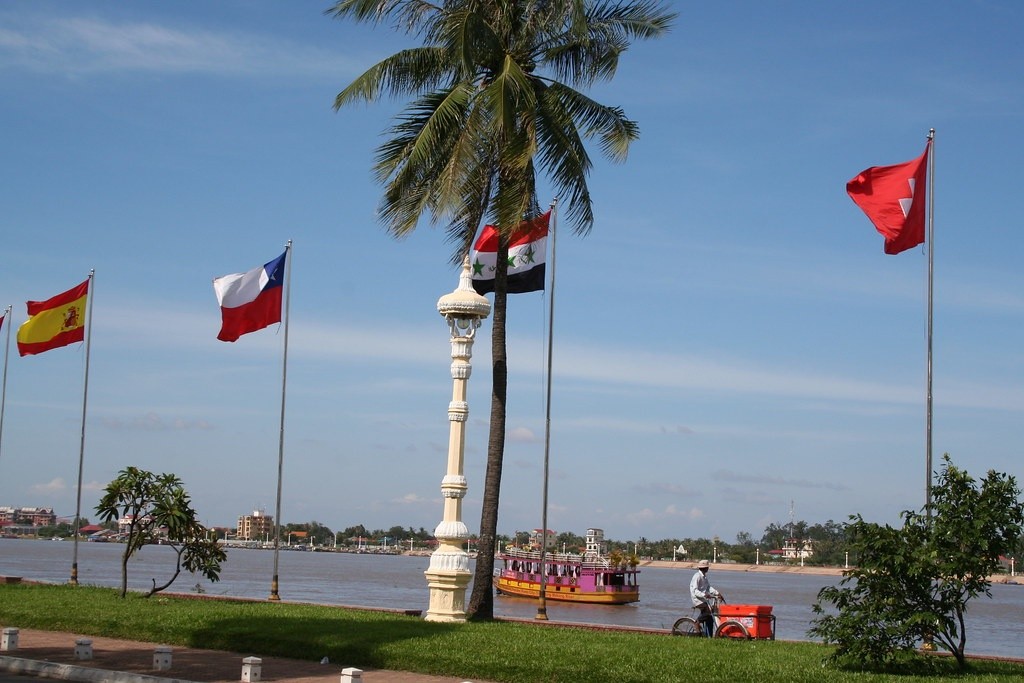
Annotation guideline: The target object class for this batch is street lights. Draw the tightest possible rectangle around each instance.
[497,540,502,553]
[385,537,387,550]
[266,533,269,543]
[563,542,566,555]
[359,536,361,548]
[334,535,337,547]
[419,256,492,635]
[310,536,313,546]
[288,533,291,547]
[713,547,717,564]
[801,549,804,567]
[673,545,676,562]
[411,537,414,552]
[225,532,227,541]
[467,539,470,553]
[756,548,759,566]
[634,544,638,556]
[845,551,849,569]
[1011,557,1015,577]
[206,530,208,540]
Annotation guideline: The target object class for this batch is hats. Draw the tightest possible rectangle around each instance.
[697,560,711,569]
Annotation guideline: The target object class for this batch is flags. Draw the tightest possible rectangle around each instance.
[213,247,288,342]
[471,206,552,297]
[846,139,930,256]
[17,278,90,357]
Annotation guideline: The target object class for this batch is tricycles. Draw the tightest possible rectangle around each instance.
[670,594,776,641]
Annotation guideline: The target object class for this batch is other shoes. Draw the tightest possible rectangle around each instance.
[694,621,701,634]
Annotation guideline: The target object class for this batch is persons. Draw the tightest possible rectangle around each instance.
[690,559,722,638]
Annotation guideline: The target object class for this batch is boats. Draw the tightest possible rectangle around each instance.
[491,542,643,605]
